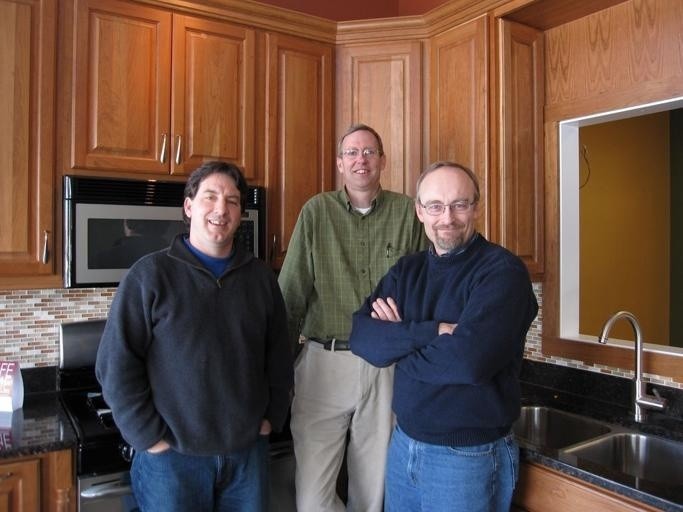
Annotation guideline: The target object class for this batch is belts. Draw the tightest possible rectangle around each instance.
[319,339,353,351]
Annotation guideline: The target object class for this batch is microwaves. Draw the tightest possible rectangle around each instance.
[61,175,267,290]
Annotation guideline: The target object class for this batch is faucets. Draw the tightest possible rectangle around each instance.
[598,309,667,422]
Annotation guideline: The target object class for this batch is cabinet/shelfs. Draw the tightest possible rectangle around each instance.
[3,0,61,290]
[264,5,541,280]
[0,391,80,512]
[57,0,264,179]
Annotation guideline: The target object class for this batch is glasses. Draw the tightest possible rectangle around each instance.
[419,201,474,216]
[342,149,382,158]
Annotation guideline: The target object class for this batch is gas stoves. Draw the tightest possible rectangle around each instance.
[59,386,136,479]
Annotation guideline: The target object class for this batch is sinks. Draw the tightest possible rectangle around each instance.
[512,405,621,452]
[563,432,683,505]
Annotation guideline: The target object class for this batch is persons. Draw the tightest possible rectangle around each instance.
[276,124,433,511]
[347,160,537,512]
[95,162,294,512]
